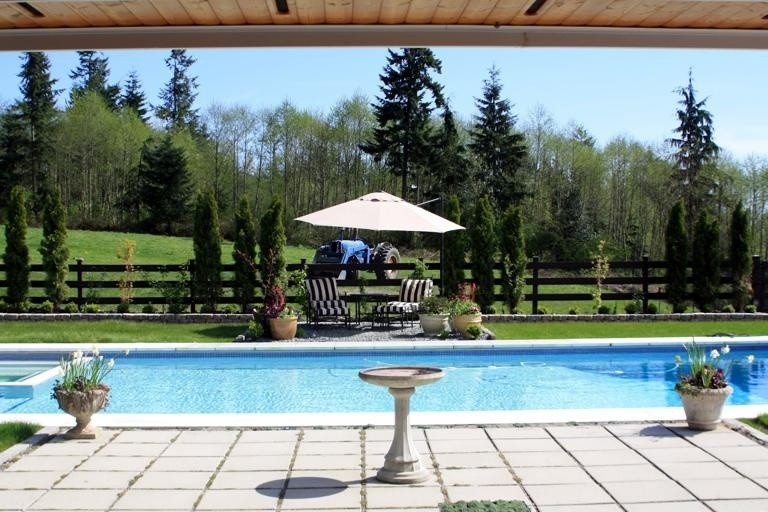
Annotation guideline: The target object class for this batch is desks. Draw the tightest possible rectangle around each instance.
[357,366,448,485]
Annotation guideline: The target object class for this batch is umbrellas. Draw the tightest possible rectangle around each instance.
[293,190,466,235]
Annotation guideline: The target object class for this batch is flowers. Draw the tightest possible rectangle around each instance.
[259,283,299,319]
[58,347,117,390]
[419,281,483,316]
[673,344,754,389]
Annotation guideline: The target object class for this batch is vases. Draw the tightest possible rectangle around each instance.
[54,385,110,440]
[417,313,482,339]
[675,382,732,431]
[253,307,300,343]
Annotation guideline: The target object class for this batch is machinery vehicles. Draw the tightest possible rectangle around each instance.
[304,228,400,280]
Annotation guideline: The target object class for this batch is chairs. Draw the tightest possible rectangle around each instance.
[304,277,435,332]
